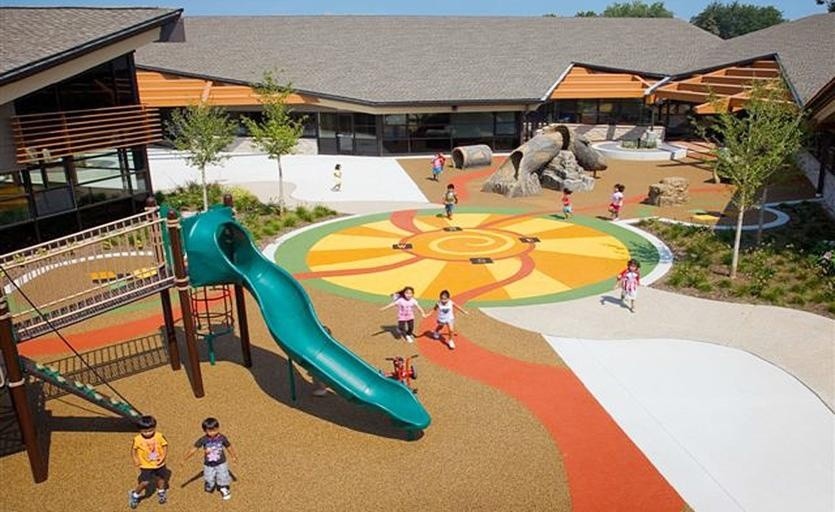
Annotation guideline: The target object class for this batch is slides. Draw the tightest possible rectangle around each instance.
[159,203,430,439]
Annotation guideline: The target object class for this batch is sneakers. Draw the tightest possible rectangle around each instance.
[609,210,620,222]
[398,334,415,343]
[447,340,458,350]
[445,210,454,220]
[432,330,441,340]
[155,489,169,507]
[207,485,232,500]
[126,489,141,511]
[564,213,575,221]
[622,298,638,313]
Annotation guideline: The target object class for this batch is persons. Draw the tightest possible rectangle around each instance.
[426,290,468,350]
[442,184,458,219]
[131,416,169,509]
[331,164,341,191]
[431,152,446,182]
[616,259,641,312]
[312,326,331,396]
[561,188,574,221]
[608,184,624,221]
[180,417,237,500]
[379,285,426,344]
